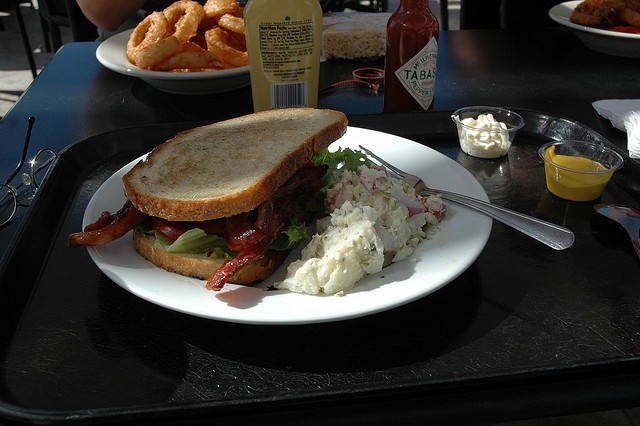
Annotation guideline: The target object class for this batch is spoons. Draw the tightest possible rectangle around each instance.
[594,202,639,260]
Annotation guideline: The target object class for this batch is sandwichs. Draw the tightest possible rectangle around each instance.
[66,105,349,292]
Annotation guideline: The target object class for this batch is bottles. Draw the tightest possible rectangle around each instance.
[384,0,438,115]
[244,0,323,113]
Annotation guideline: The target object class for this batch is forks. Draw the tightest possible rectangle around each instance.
[354,144,575,251]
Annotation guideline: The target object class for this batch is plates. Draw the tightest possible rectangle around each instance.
[83,126,494,327]
[548,0,640,59]
[96,26,250,95]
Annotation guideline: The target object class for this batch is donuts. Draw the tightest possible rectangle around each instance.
[126,0,249,73]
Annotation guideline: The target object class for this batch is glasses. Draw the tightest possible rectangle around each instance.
[0,116,57,226]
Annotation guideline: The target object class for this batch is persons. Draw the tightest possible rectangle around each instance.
[75,0,184,41]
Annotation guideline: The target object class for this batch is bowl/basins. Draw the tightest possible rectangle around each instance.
[451,106,524,159]
[538,140,625,201]
